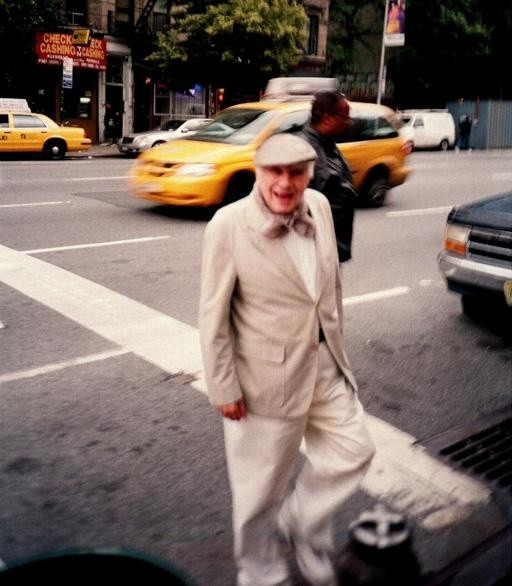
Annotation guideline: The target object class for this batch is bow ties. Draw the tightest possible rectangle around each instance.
[261,213,318,241]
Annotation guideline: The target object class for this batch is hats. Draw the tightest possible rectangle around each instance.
[254,134,318,170]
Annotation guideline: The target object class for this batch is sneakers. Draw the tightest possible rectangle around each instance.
[278,515,335,584]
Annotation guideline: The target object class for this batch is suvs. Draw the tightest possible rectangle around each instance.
[438,191,512,323]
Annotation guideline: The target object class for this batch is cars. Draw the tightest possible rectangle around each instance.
[1,95,92,161]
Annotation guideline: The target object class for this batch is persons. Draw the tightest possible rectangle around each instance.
[191,128,379,586]
[101,101,119,148]
[289,89,362,353]
